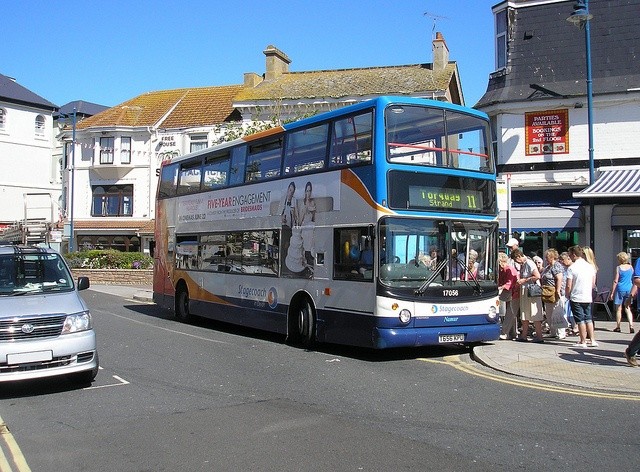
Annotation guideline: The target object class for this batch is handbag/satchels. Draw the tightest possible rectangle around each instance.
[528,277,542,297]
[499,289,513,302]
[541,284,556,303]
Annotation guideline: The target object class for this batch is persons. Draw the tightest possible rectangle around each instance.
[505,237,520,252]
[532,255,545,274]
[565,245,599,347]
[480,251,487,270]
[559,252,581,337]
[359,231,388,265]
[540,248,566,341]
[276,182,298,255]
[624,256,640,367]
[460,254,479,280]
[497,252,521,339]
[409,245,460,279]
[510,248,544,343]
[457,245,467,264]
[575,246,598,344]
[608,252,638,333]
[468,249,480,270]
[298,181,316,251]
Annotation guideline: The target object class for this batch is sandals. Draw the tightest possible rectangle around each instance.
[614,328,620,332]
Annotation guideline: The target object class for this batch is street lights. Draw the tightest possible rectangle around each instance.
[566,0,595,252]
[57,108,77,254]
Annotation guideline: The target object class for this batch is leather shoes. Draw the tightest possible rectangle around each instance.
[624,349,638,367]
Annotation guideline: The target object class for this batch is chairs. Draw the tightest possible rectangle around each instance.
[592,288,614,320]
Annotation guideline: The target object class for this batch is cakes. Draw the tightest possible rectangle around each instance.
[285,227,307,272]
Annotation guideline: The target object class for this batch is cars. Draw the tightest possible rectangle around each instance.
[0,246,98,383]
[175,241,198,256]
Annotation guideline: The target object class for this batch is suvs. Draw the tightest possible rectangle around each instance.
[200,262,276,276]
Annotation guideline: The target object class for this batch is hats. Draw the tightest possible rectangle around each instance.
[506,238,519,247]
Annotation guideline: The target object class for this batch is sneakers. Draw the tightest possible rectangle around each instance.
[499,334,512,339]
[587,342,599,347]
[572,343,587,348]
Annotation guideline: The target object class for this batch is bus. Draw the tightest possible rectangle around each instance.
[153,96,524,350]
